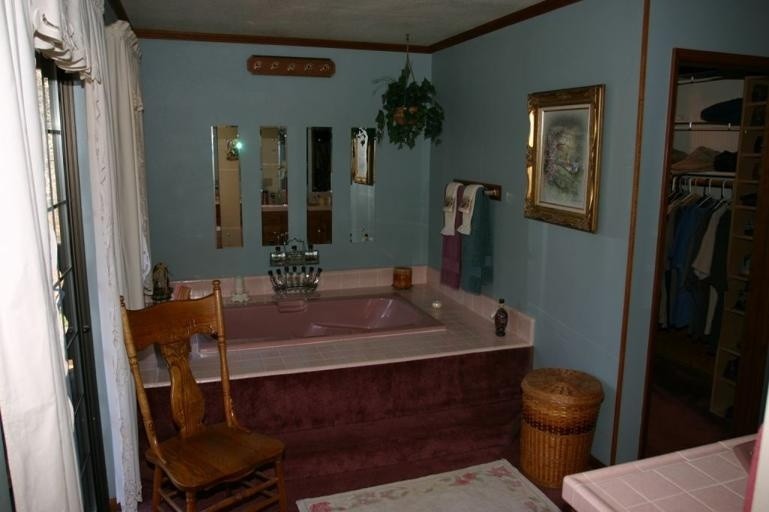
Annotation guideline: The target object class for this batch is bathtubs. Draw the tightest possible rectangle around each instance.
[196,292,448,355]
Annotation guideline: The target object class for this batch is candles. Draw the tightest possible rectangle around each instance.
[119,279,285,512]
[233,275,248,292]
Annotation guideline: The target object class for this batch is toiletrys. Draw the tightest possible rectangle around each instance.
[268,245,323,294]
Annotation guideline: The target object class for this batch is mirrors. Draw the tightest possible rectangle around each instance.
[209,123,333,250]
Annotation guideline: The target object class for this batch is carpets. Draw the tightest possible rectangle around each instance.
[293,458,562,512]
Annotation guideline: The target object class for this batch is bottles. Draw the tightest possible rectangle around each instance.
[269,244,319,266]
[268,266,323,294]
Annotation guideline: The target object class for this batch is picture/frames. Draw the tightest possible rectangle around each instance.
[523,84,606,235]
[352,130,373,187]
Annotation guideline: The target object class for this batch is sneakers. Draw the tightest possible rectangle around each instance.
[671,146,721,174]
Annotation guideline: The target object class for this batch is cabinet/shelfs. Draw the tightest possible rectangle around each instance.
[707,75,767,425]
[668,121,739,189]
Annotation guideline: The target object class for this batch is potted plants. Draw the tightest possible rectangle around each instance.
[368,29,445,148]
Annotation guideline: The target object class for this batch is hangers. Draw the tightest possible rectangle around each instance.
[660,172,734,221]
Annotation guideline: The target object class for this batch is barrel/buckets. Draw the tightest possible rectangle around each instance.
[393,267,411,289]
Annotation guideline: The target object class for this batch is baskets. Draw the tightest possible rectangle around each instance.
[520,368,604,488]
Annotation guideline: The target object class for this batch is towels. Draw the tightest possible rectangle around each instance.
[436,182,487,290]
